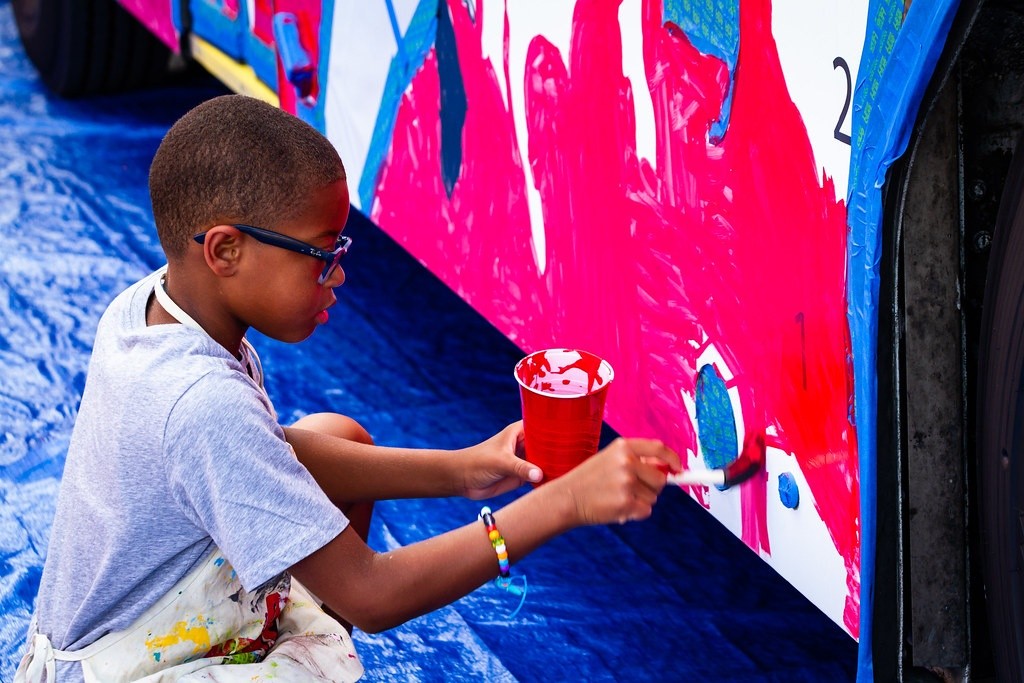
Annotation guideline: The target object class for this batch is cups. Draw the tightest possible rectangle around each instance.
[514,347,615,490]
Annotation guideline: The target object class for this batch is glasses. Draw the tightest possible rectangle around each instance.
[194,225,352,285]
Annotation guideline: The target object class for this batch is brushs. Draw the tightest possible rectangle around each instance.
[665,446,760,488]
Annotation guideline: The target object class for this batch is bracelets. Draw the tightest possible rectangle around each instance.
[482,505,511,576]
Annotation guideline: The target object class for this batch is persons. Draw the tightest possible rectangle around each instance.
[14,93,684,683]
